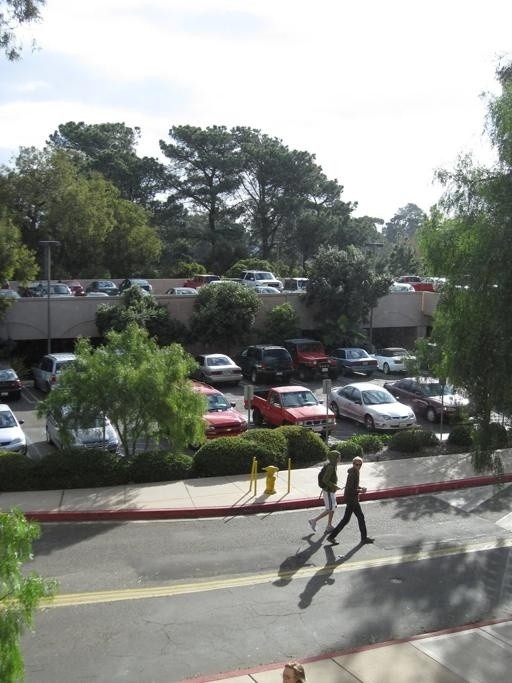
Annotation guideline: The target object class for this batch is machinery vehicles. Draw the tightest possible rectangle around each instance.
[369,346,417,373]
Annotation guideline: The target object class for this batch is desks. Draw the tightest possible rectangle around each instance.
[363,242,384,344]
[38,239,62,352]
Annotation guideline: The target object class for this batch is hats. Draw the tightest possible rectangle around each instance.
[324,381,416,432]
[382,374,475,422]
[44,400,120,454]
[0,360,22,400]
[0,402,27,455]
[193,352,244,386]
[328,347,379,376]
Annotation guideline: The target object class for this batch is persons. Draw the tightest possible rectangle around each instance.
[307,450,341,532]
[326,455,376,543]
[282,662,307,683]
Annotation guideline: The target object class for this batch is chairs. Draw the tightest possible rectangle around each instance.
[326,525,335,532]
[326,535,339,544]
[362,537,375,543]
[308,518,316,531]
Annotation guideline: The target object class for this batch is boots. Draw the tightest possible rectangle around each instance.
[234,344,295,383]
[184,377,248,449]
[32,352,88,392]
[283,338,338,381]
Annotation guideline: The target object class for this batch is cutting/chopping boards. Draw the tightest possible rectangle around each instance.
[262,466,280,494]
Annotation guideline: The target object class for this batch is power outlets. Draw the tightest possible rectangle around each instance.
[244,385,337,436]
